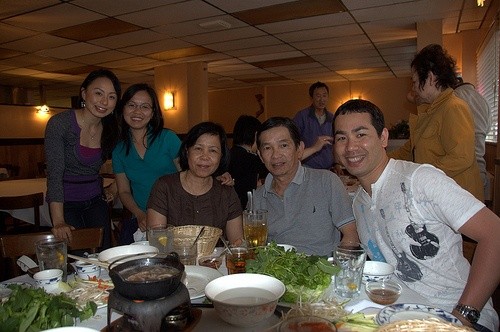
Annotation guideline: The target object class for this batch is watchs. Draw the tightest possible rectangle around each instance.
[456,305,480,323]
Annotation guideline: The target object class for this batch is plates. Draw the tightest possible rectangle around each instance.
[280,314,338,332]
[267,243,298,253]
[376,302,464,328]
[97,243,159,263]
[180,264,223,300]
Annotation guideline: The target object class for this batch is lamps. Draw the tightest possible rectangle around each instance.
[37,103,53,115]
[163,89,175,111]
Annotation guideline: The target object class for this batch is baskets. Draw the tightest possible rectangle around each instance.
[166,225,223,256]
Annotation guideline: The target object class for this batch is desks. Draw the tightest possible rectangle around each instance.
[0,178,54,227]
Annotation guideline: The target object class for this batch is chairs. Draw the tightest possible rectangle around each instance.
[0,191,44,235]
[0,226,105,257]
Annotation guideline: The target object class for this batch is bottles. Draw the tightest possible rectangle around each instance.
[148,224,173,254]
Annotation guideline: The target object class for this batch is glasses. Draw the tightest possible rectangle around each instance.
[125,100,154,114]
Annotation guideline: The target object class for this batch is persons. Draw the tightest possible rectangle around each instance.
[245,117,359,257]
[292,82,335,169]
[407,44,491,206]
[332,99,500,332]
[44,69,122,257]
[227,115,269,212]
[111,83,182,246]
[146,121,245,248]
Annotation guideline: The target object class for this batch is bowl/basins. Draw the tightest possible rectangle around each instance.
[108,251,185,301]
[363,260,395,281]
[33,268,63,288]
[204,274,286,326]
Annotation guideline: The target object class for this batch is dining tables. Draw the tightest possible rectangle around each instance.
[0,247,493,332]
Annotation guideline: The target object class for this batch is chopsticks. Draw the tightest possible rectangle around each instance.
[66,252,116,270]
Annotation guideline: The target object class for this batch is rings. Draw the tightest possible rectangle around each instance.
[324,139,326,141]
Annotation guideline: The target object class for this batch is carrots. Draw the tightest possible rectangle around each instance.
[90,276,112,302]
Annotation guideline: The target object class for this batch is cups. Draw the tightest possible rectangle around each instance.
[175,244,198,265]
[333,241,367,298]
[37,238,68,274]
[242,208,269,247]
[199,256,222,270]
[224,248,249,273]
[366,280,403,304]
[69,256,101,279]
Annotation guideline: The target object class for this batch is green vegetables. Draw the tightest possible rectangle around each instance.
[245,239,339,306]
[232,257,244,263]
[0,283,98,332]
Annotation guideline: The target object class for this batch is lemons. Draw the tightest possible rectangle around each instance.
[158,236,167,248]
[56,251,63,265]
